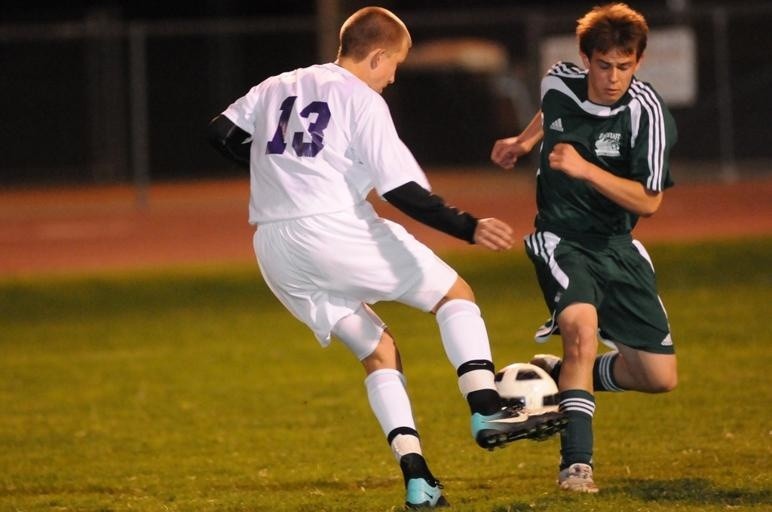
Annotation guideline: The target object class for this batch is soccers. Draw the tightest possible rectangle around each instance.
[495,363,559,411]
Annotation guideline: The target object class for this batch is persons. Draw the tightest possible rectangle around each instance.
[490,2,679,492]
[205,6,569,511]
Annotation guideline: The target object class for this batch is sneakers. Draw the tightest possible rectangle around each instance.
[529,353,561,376]
[470,401,561,451]
[405,478,449,510]
[557,456,599,494]
